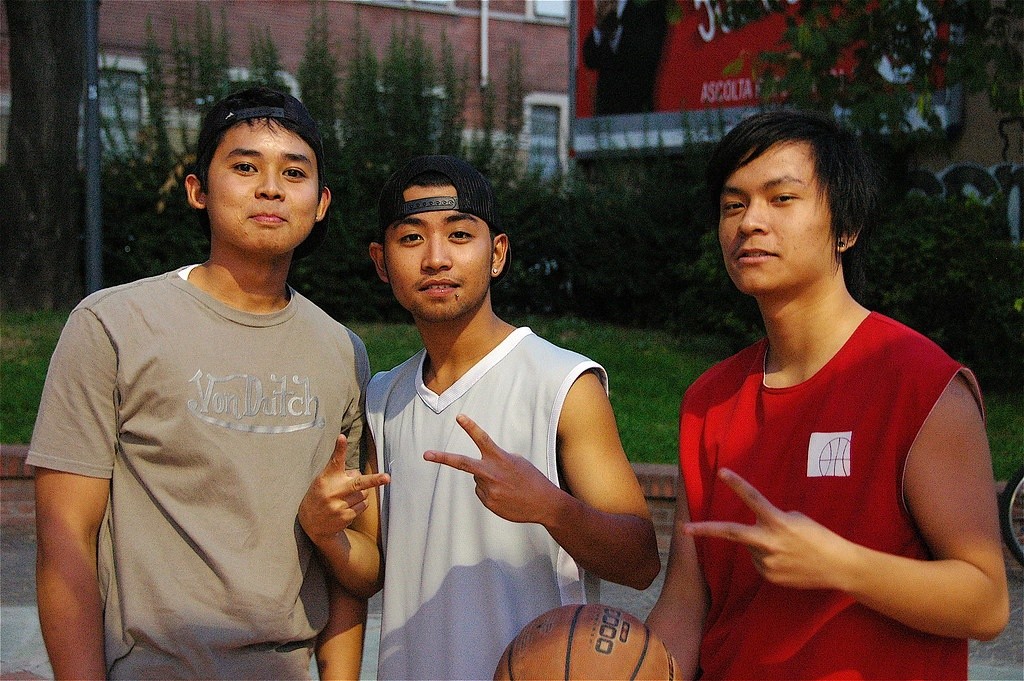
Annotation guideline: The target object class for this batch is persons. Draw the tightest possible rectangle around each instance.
[23,86,373,681]
[642,112,1013,681]
[580,1,669,117]
[296,153,662,681]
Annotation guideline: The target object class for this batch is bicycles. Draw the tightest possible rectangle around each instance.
[999,463,1024,566]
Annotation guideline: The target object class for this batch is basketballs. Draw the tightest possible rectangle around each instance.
[492,603,681,681]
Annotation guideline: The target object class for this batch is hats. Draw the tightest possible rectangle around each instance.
[198,88,329,260]
[379,155,511,287]
